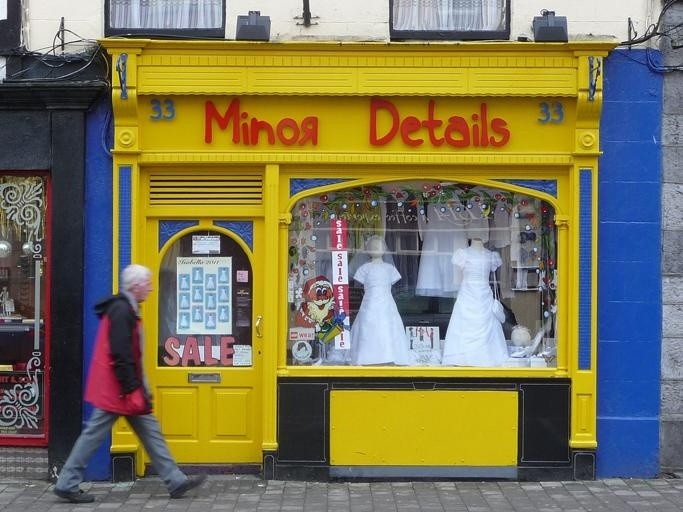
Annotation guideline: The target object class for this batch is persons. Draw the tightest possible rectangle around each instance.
[54,264,207,503]
[452,239,502,364]
[0,286,14,316]
[352,235,401,363]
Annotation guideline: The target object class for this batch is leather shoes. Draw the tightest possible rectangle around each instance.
[170,473,206,500]
[52,489,99,504]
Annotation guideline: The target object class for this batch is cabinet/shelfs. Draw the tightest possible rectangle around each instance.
[508,193,541,345]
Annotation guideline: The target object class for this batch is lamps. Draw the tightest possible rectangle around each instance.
[533,11,568,42]
[236,11,271,41]
